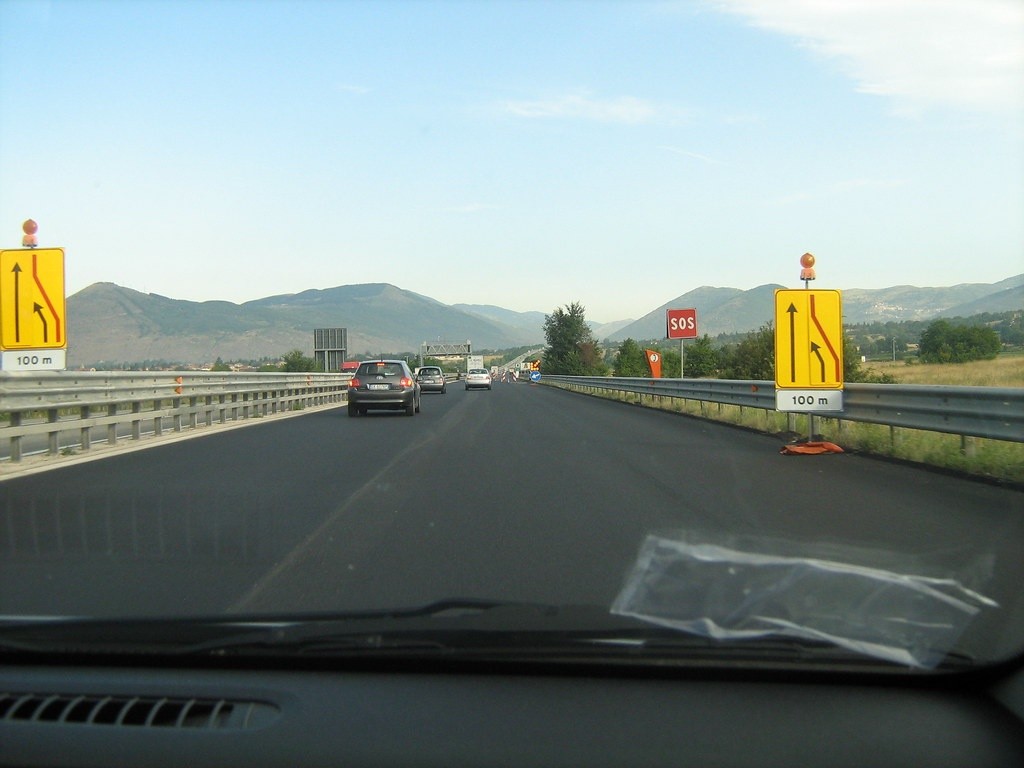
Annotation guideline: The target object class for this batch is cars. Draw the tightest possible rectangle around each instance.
[463,368,492,391]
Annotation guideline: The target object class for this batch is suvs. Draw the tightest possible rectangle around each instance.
[347,360,422,416]
[416,365,447,394]
